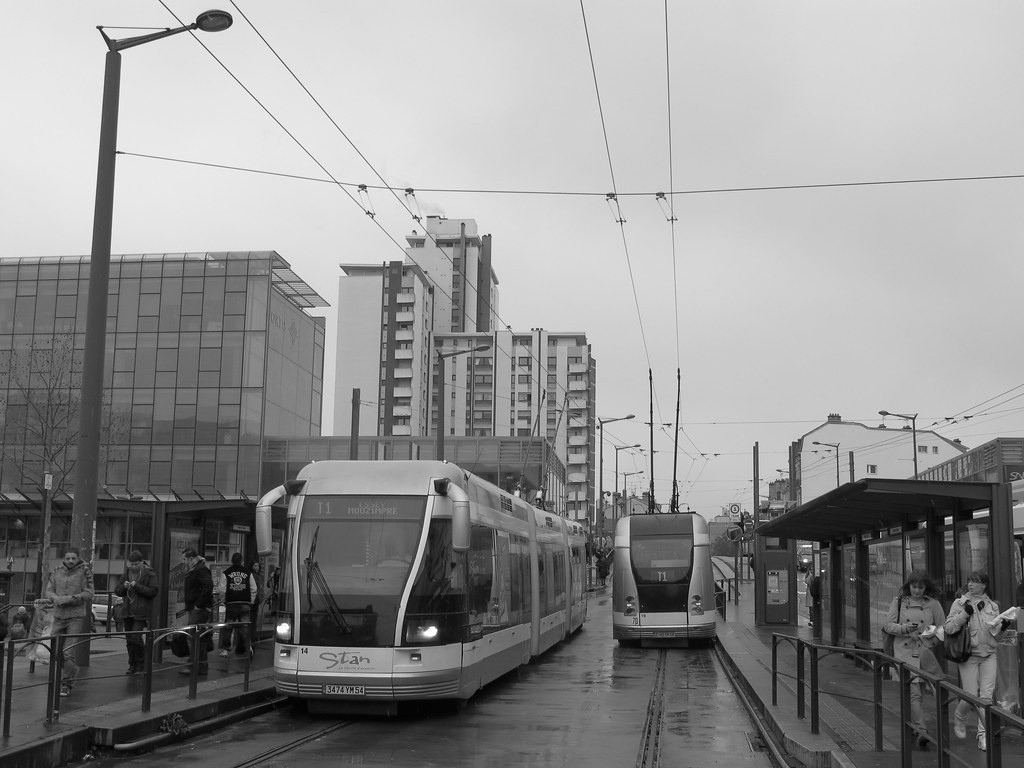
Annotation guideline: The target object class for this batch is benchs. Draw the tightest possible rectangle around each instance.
[839,638,889,679]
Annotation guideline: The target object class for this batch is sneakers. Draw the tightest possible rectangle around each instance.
[953,714,966,740]
[976,732,986,751]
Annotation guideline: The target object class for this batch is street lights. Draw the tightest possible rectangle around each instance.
[614,444,641,519]
[812,441,841,488]
[879,410,918,478]
[72,6,235,671]
[597,414,635,556]
[622,471,645,515]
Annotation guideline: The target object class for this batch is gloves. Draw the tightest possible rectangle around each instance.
[1001,619,1010,632]
[964,603,974,615]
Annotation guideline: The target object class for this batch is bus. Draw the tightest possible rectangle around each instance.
[610,512,719,647]
[254,459,589,711]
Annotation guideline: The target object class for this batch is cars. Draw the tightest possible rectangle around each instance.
[797,544,813,570]
[86,595,120,624]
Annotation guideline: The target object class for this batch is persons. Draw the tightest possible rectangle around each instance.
[114,550,159,675]
[804,564,814,627]
[90,612,97,634]
[376,532,414,567]
[945,571,1011,751]
[883,568,946,747]
[12,606,31,644]
[178,548,214,681]
[47,548,94,696]
[218,553,261,657]
[585,535,614,585]
[113,597,125,632]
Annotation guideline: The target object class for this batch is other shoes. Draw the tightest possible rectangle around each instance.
[178,667,209,676]
[220,649,228,658]
[912,729,930,748]
[808,621,814,627]
[60,686,69,696]
[126,664,145,674]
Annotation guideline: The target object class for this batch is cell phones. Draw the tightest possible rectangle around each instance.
[912,623,918,628]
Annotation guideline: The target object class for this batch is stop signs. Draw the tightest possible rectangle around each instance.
[745,520,754,528]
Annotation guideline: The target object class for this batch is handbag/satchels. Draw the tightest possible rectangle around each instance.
[882,627,896,658]
[942,602,973,663]
[170,626,214,658]
